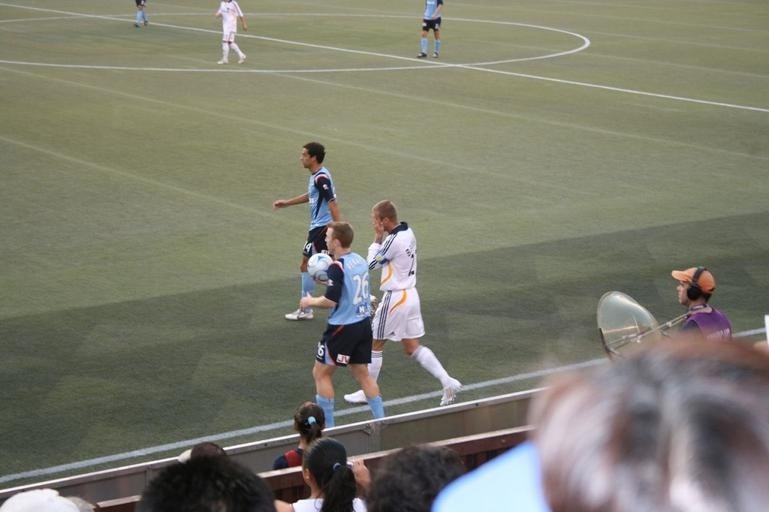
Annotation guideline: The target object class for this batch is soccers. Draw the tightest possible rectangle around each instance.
[307,253,333,281]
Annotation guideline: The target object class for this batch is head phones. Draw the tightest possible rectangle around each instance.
[688,266,712,300]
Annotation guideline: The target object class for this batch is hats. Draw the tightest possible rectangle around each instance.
[671,265,716,297]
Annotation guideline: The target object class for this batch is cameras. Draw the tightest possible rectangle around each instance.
[346,462,356,474]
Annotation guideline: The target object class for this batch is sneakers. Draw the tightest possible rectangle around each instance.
[217,60,229,64]
[415,52,427,57]
[283,308,314,321]
[237,55,247,64]
[432,50,439,58]
[342,389,368,406]
[439,379,464,408]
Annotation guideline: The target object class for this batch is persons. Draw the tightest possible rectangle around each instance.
[215,1,248,65]
[297,223,388,425]
[671,266,733,343]
[274,144,377,324]
[134,1,148,28]
[341,197,463,408]
[417,0,444,60]
